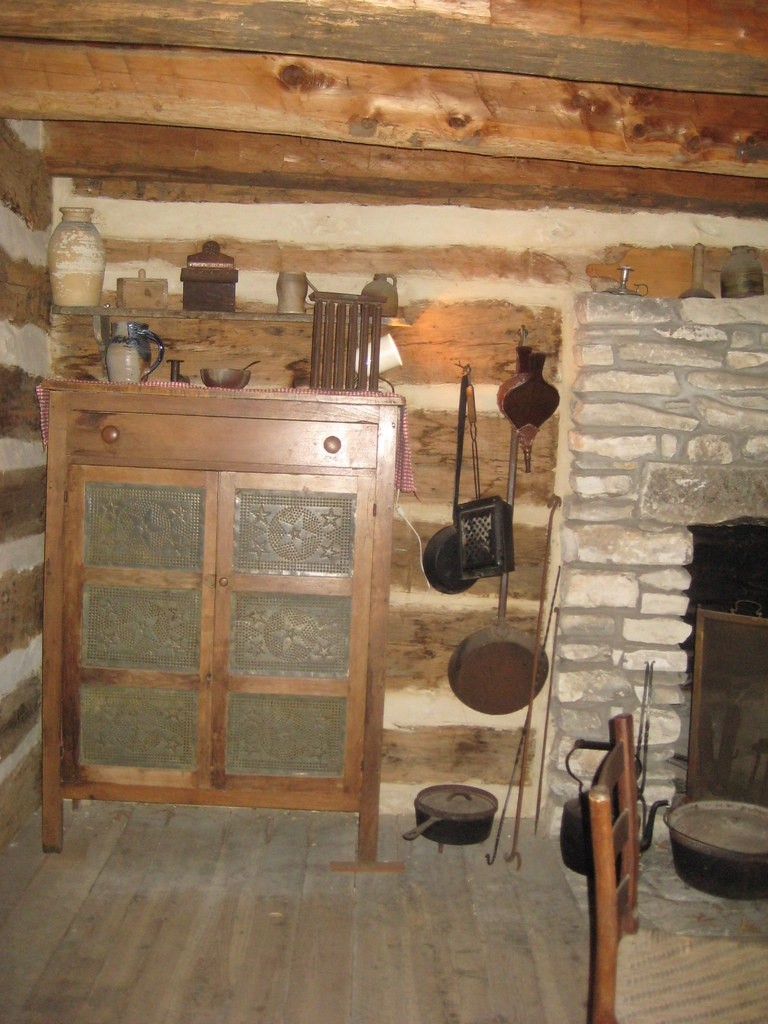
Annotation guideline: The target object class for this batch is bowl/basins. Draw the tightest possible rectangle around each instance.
[200,368,251,389]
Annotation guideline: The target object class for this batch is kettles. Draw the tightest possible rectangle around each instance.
[559,739,669,876]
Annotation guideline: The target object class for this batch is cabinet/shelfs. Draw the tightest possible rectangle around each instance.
[39,381,404,866]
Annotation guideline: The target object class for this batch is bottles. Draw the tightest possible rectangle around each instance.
[47,207,106,307]
[361,273,398,317]
[275,271,308,314]
[720,245,764,299]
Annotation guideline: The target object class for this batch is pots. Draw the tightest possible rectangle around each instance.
[402,783,498,846]
[663,799,768,902]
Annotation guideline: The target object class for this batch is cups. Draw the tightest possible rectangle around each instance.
[355,333,402,378]
[104,321,165,383]
